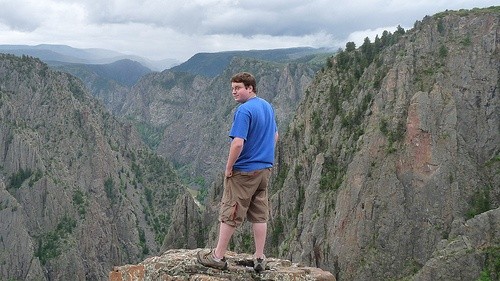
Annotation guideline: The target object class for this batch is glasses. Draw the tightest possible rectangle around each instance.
[231,85,246,90]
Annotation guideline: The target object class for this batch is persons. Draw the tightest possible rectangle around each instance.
[196,73,279,272]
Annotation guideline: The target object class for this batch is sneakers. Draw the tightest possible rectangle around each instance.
[197,248,227,270]
[253,253,266,271]
